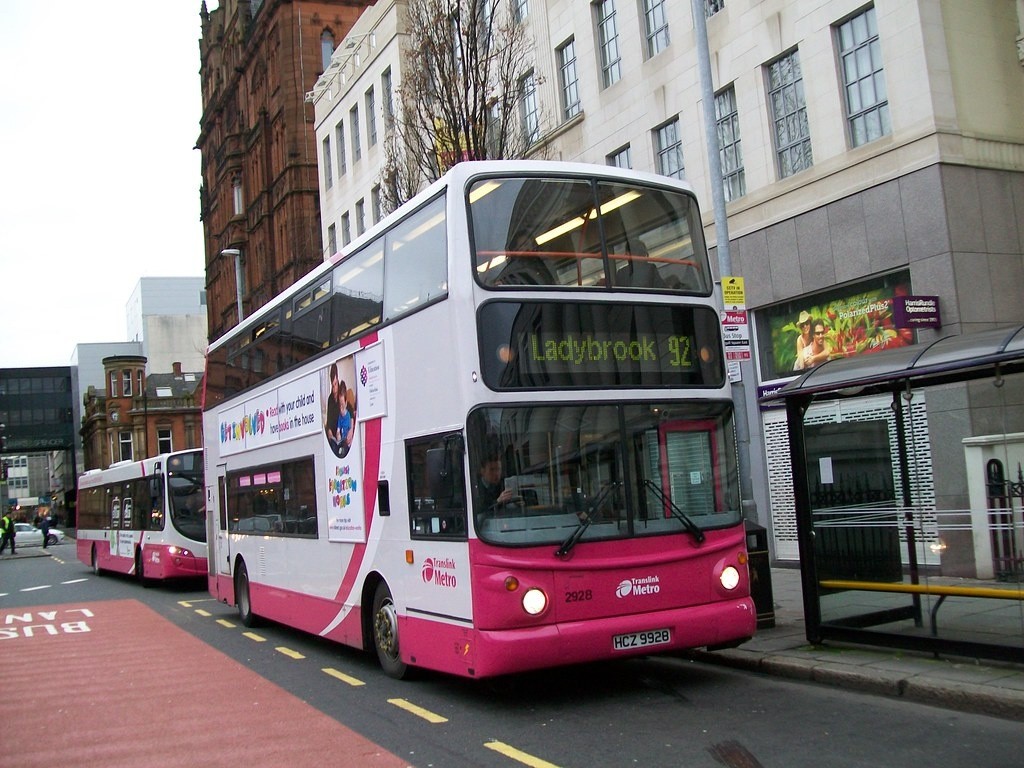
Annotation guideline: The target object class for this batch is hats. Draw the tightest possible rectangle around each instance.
[796,311,813,327]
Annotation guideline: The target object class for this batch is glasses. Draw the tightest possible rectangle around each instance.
[814,332,824,335]
[800,320,809,328]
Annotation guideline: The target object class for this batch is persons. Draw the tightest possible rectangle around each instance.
[326,364,354,457]
[616,239,667,287]
[793,311,831,372]
[0,512,18,554]
[492,230,561,285]
[473,455,525,530]
[14,512,50,550]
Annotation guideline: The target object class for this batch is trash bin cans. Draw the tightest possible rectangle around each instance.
[744,520,776,629]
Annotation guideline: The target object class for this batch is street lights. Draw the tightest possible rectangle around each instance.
[219,247,245,330]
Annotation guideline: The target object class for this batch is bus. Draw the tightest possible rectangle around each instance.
[76,448,207,584]
[200,160,760,682]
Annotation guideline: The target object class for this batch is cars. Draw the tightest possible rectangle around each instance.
[44,514,59,527]
[4,523,66,547]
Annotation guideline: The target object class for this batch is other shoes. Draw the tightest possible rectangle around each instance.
[11,552,17,555]
